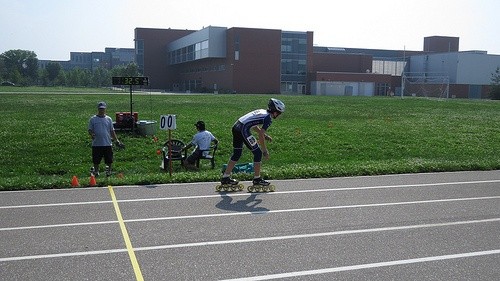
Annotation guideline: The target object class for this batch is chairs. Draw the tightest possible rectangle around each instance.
[163,139,187,169]
[197,140,219,169]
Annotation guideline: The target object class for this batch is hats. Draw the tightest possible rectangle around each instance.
[98,102,106,109]
[194,121,205,128]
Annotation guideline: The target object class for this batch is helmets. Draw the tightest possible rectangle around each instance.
[269,97,285,113]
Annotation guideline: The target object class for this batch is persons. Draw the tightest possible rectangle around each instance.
[88,102,120,177]
[177,121,218,171]
[216,98,285,192]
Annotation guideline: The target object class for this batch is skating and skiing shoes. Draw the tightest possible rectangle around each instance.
[247,175,275,192]
[215,176,244,192]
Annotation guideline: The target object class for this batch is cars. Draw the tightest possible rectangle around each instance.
[1,82,15,86]
